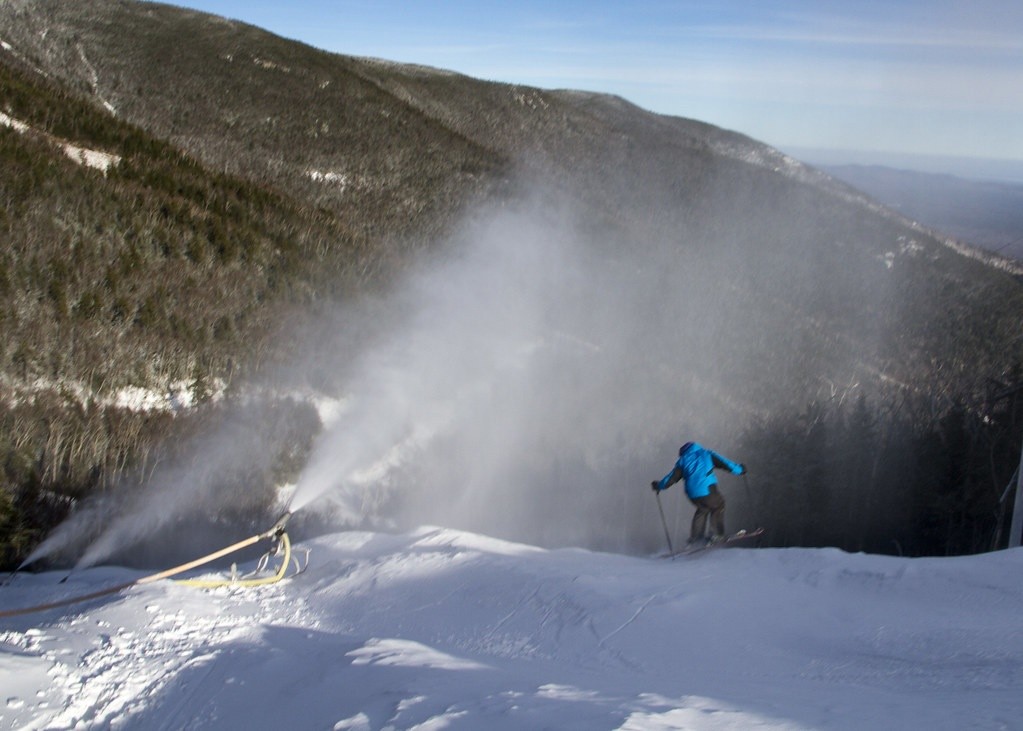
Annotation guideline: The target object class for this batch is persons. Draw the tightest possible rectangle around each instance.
[653,442,748,548]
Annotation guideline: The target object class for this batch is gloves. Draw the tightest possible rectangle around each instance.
[651,480,661,495]
[740,464,747,474]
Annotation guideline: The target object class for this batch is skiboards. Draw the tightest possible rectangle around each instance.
[639,526,766,558]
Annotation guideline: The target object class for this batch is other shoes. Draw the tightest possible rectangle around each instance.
[709,535,729,543]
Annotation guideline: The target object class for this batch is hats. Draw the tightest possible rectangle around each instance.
[679,442,693,456]
